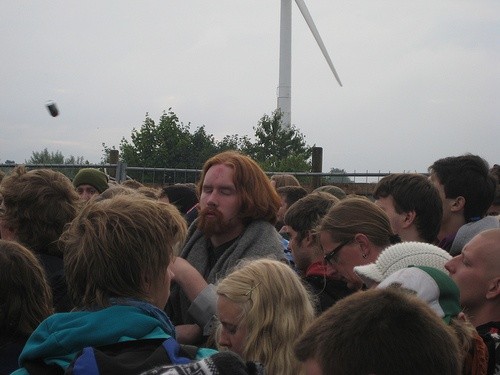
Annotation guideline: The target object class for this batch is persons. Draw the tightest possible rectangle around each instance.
[0,150,500,375]
[292,287,461,375]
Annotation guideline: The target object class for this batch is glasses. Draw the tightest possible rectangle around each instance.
[324,241,346,265]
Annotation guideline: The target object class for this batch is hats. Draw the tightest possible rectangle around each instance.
[71,167,110,193]
[373,264,462,326]
[449,215,499,256]
[164,186,197,214]
[354,242,454,289]
